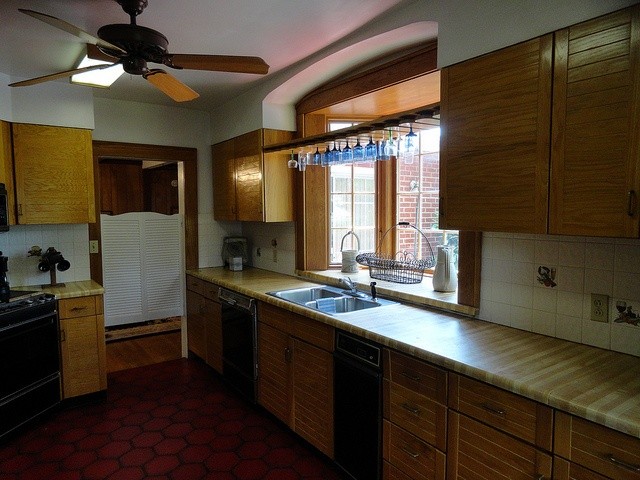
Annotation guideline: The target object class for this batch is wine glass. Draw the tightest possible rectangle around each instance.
[365,136,376,159]
[384,128,397,157]
[314,147,321,164]
[354,139,364,159]
[307,147,314,165]
[324,145,331,161]
[396,124,405,159]
[298,149,306,172]
[288,150,297,169]
[343,141,352,160]
[379,141,389,158]
[406,127,417,148]
[331,143,338,161]
[403,133,413,165]
[338,145,342,160]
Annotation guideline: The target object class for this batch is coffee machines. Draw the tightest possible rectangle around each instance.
[224,236,251,271]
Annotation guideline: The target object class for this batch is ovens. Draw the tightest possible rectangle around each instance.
[217,287,259,402]
[0,294,60,441]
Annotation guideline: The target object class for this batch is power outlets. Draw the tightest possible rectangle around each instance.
[589,294,609,323]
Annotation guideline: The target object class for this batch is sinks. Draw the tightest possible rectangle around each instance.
[306,297,382,314]
[275,288,343,304]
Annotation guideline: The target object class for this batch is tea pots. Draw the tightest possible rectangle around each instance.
[432,245,457,292]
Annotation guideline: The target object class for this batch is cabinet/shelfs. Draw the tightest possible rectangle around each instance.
[552,408,639,479]
[381,349,448,478]
[10,123,96,225]
[447,374,555,480]
[549,2,640,240]
[0,117,16,230]
[439,33,553,235]
[210,129,295,224]
[254,302,334,459]
[58,295,109,400]
[185,273,223,372]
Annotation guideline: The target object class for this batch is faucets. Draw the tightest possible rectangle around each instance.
[338,277,357,294]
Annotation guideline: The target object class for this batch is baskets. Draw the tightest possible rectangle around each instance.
[341,230,360,274]
[355,222,436,285]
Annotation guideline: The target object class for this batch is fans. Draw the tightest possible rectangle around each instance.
[9,1,269,103]
